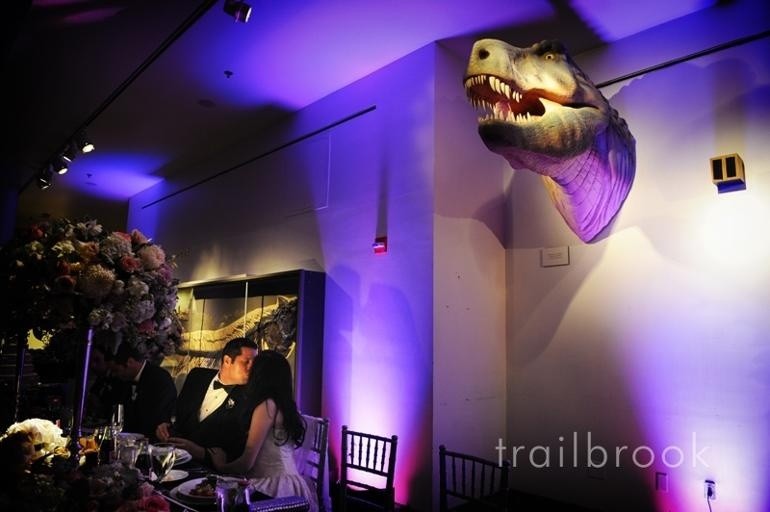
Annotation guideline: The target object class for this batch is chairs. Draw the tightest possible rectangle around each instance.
[176,367,219,431]
[339,426,397,512]
[292,415,328,512]
[439,444,510,512]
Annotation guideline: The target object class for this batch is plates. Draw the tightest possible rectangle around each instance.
[168,447,249,500]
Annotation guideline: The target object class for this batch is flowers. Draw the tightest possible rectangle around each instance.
[0,214,183,355]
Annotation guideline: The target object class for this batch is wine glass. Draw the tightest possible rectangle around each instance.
[151,442,175,484]
[111,405,122,457]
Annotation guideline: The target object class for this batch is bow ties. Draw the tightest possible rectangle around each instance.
[213,381,233,393]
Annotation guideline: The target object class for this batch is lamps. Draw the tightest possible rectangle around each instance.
[38,0,252,191]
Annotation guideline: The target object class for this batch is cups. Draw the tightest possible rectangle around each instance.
[221,485,251,512]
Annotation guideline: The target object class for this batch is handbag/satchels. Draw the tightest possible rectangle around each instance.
[250,496,309,512]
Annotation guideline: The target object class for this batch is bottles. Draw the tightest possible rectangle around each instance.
[135,438,157,476]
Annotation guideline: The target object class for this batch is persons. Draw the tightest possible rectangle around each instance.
[31,329,178,435]
[154,338,259,471]
[205,349,321,512]
